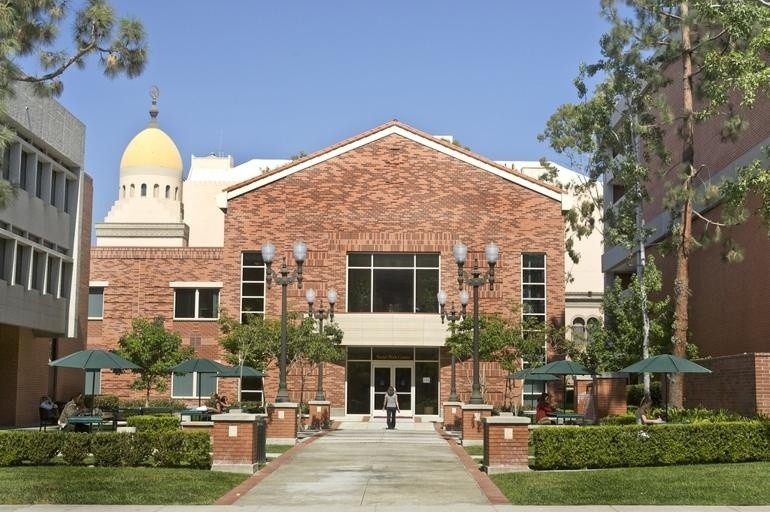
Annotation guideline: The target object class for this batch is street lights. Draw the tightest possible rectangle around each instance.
[306,287,338,400]
[436,289,470,402]
[261,240,307,403]
[453,238,500,405]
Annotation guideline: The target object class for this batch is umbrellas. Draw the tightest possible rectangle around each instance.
[502,360,603,425]
[47,349,144,433]
[162,358,271,408]
[617,354,714,421]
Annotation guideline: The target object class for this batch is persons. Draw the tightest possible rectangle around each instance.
[382,386,400,430]
[40,396,60,419]
[207,393,231,414]
[57,392,89,434]
[635,396,666,439]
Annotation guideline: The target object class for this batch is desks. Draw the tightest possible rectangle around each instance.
[553,413,585,424]
[68,417,104,432]
[180,410,209,421]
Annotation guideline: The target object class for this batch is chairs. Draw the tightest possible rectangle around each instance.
[38,401,64,432]
